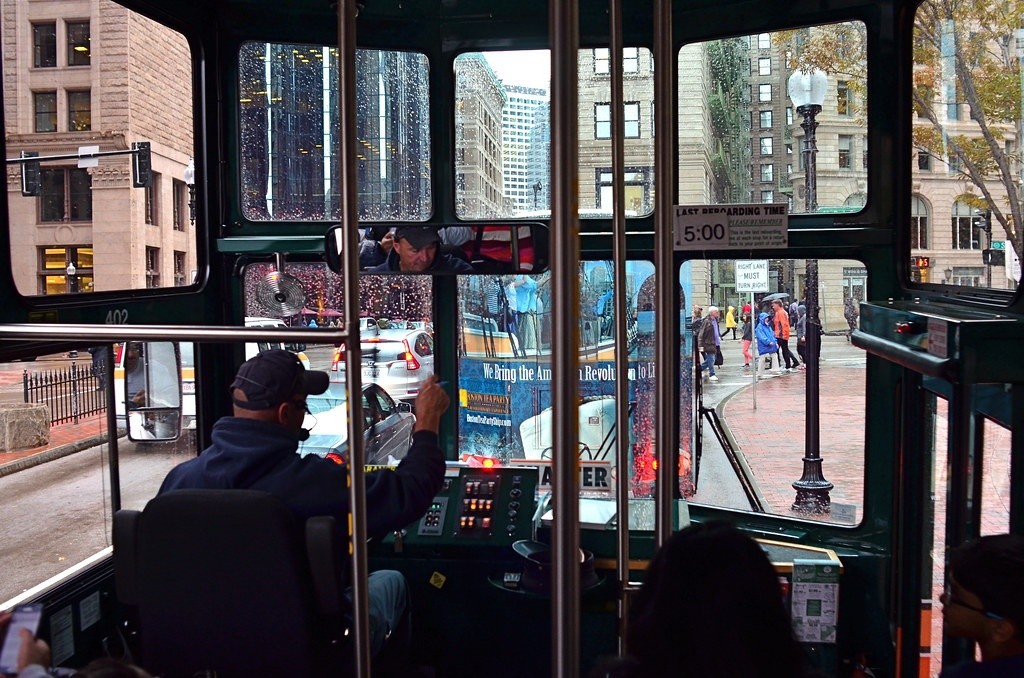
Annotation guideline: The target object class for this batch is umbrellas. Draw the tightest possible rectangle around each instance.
[761,292,789,304]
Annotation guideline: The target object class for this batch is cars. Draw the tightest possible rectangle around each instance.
[295,380,417,468]
[358,318,431,332]
[328,330,436,402]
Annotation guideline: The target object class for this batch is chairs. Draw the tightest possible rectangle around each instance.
[109,493,340,678]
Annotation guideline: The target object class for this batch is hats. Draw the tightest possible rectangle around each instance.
[226,347,330,410]
[395,226,441,248]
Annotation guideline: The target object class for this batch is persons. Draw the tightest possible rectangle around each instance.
[784,301,789,313]
[796,305,807,370]
[156,350,449,678]
[790,299,800,330]
[740,301,751,332]
[845,297,859,341]
[485,275,551,349]
[436,224,535,273]
[771,298,800,369]
[939,535,1024,678]
[367,225,475,273]
[0,610,52,678]
[692,304,723,381]
[585,519,830,678]
[596,283,614,343]
[738,311,759,368]
[721,306,738,340]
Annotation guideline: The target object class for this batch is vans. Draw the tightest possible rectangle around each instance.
[112,315,310,448]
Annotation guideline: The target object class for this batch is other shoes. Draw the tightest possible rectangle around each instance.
[741,364,750,368]
[771,371,783,378]
[709,375,719,381]
[757,375,767,380]
[782,368,793,372]
[791,364,801,369]
[846,334,850,342]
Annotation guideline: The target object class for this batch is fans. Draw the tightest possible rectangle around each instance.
[256,252,307,318]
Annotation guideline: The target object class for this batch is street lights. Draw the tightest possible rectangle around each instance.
[785,52,835,516]
[65,263,78,358]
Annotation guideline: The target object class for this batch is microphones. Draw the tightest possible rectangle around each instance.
[297,415,318,441]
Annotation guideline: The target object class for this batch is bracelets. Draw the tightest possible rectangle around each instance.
[611,287,614,289]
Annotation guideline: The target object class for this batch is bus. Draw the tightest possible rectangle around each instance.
[457,209,705,535]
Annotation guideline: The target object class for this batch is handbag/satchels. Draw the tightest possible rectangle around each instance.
[713,345,723,365]
[844,302,854,319]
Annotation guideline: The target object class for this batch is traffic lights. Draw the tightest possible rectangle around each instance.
[915,256,929,269]
[973,207,992,232]
